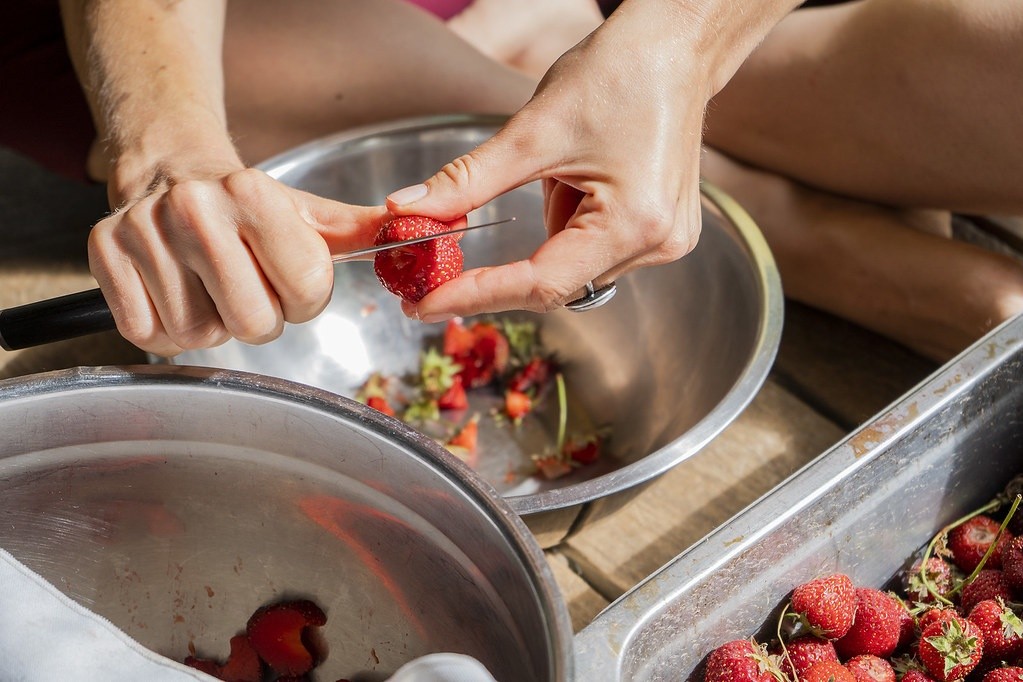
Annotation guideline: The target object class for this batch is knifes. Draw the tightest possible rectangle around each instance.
[0,217,517,351]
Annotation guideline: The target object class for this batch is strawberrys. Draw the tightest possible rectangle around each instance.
[185,599,328,682]
[701,514,1023,682]
[373,215,465,304]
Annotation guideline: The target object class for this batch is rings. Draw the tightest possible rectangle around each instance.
[562,280,616,313]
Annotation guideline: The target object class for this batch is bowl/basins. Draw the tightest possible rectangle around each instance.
[0,364,576,682]
[145,111,786,514]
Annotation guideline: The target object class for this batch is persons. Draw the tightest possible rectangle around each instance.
[55,0,1023,368]
